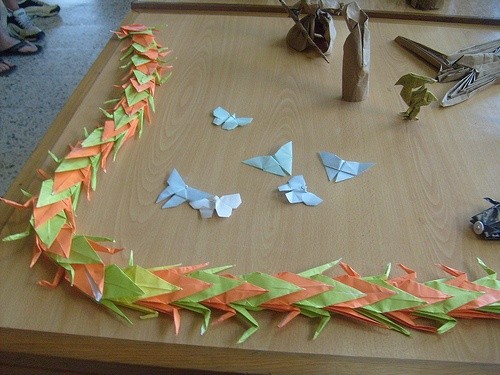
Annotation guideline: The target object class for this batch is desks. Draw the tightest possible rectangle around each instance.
[0,0,500,374]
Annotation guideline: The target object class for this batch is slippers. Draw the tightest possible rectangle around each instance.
[0,58,17,76]
[0,39,42,56]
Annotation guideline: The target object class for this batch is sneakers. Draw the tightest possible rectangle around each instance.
[6,8,45,42]
[18,0,60,17]
